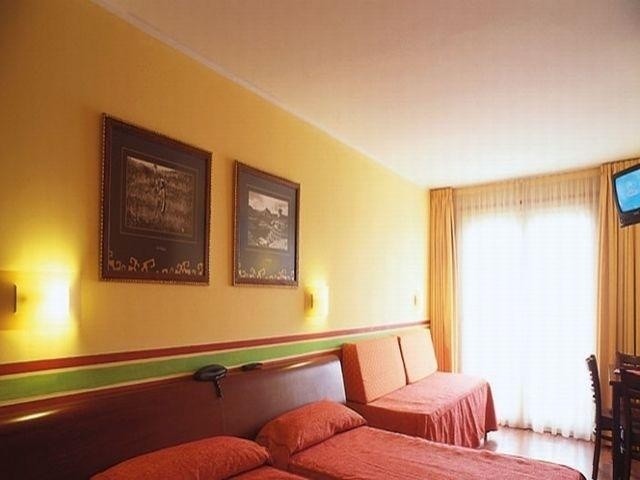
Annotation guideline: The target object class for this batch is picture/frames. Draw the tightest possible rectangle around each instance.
[231,160,302,289]
[96,113,214,287]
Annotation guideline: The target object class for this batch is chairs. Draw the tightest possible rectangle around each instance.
[586,350,640,480]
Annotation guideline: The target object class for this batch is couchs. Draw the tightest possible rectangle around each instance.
[339,325,501,447]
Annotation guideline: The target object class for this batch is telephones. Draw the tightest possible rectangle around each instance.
[194,364,228,381]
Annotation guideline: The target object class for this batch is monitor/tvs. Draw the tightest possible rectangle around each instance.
[612,163,640,227]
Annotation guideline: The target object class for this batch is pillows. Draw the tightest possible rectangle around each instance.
[90,434,272,479]
[256,399,367,456]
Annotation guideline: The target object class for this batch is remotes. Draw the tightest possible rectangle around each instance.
[239,363,263,371]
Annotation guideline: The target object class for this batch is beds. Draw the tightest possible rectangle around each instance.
[0,355,584,479]
